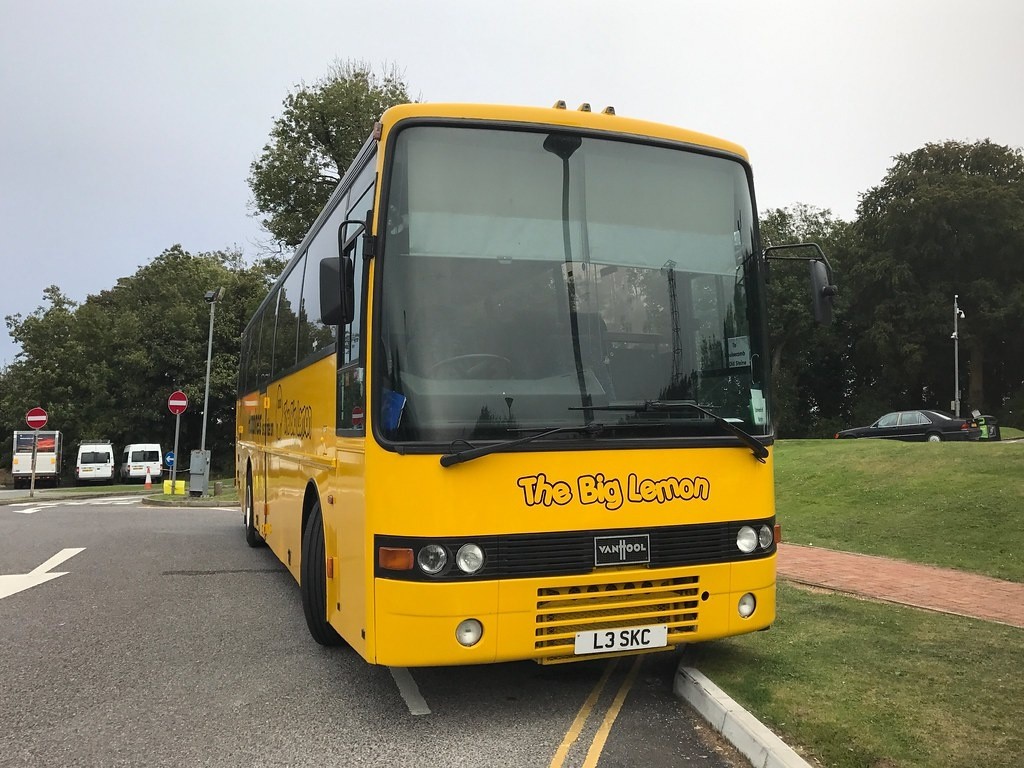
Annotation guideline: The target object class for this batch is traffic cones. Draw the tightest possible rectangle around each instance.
[144,467,152,489]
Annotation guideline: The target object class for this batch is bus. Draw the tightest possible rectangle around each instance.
[233,100,784,675]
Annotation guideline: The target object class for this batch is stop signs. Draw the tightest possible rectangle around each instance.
[352,406,363,426]
[167,391,188,414]
[25,408,48,429]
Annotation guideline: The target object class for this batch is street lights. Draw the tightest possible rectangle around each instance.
[189,286,227,497]
[949,294,960,417]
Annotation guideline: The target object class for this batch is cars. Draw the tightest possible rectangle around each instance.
[835,409,981,442]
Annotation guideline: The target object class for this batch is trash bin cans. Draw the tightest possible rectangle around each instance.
[977,414,1001,442]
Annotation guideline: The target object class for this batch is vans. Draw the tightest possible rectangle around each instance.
[12,430,63,489]
[119,443,163,484]
[75,439,115,486]
